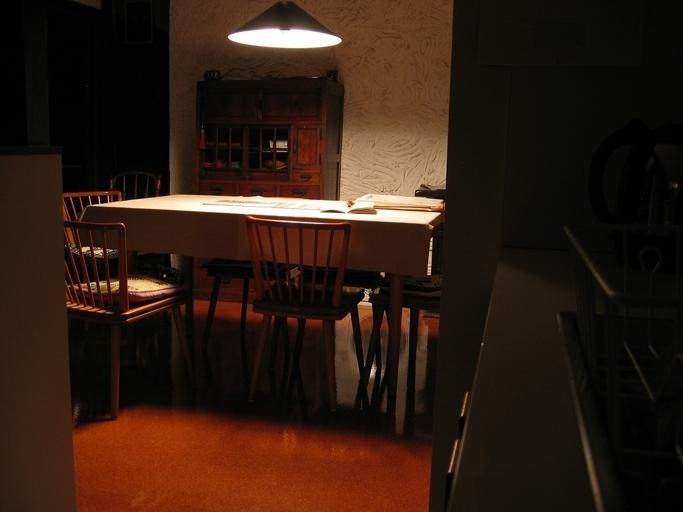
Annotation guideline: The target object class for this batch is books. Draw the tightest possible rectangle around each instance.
[320,200,375,214]
[230,161,241,168]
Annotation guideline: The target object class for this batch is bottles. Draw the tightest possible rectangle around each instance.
[325,48,338,82]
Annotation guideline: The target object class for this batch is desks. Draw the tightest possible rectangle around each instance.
[445,247,683,512]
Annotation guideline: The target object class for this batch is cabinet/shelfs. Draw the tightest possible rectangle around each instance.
[192,80,345,305]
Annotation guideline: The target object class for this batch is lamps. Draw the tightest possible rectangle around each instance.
[227,0,342,48]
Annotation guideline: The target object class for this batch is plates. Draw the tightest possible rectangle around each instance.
[231,161,240,168]
